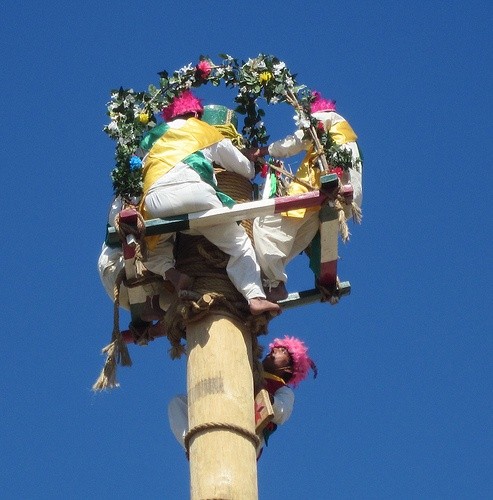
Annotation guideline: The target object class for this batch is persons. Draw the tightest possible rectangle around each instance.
[139,90,280,315]
[247,91,363,301]
[168,336,318,452]
[96,192,192,324]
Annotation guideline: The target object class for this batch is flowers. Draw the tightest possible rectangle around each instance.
[103,53,364,202]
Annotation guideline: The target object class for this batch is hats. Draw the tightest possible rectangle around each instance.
[310,90,336,113]
[162,88,203,121]
[268,335,311,389]
